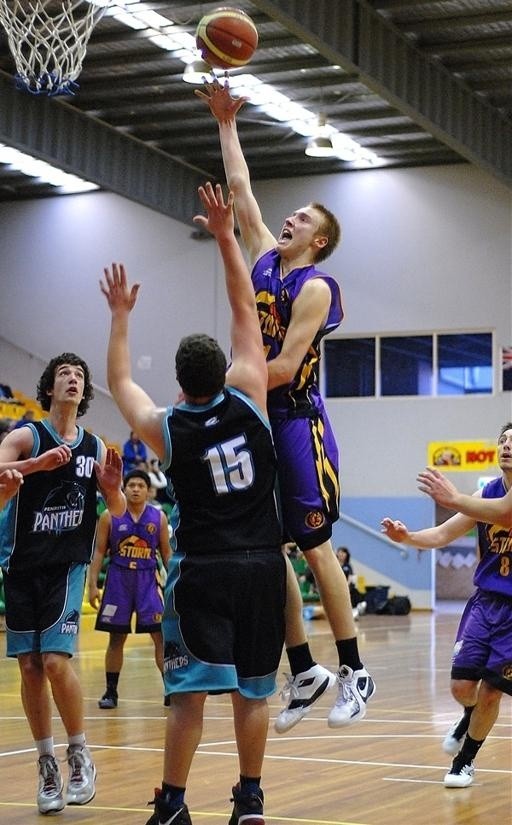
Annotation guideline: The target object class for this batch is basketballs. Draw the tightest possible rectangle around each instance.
[193,5,259,69]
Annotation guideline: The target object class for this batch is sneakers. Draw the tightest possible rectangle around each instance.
[34,753,65,815]
[63,743,98,806]
[274,663,338,734]
[97,688,118,709]
[144,787,192,824]
[443,752,475,789]
[227,780,267,824]
[328,664,379,729]
[442,714,470,756]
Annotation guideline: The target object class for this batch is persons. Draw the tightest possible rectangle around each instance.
[415,466,512,534]
[191,65,378,734]
[98,180,286,825]
[0,352,128,816]
[0,468,25,511]
[1,385,38,441]
[288,536,375,622]
[112,430,175,506]
[89,472,175,709]
[378,421,511,790]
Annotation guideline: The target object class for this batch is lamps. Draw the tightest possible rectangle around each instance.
[304,110,333,157]
[182,57,213,86]
